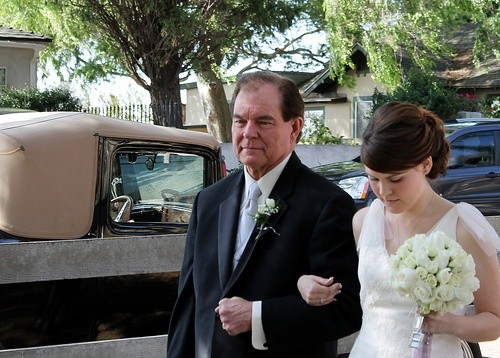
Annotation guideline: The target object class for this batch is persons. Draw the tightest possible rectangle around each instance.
[165,71,360,358]
[297,100,500,358]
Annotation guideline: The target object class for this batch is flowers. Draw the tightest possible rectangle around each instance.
[388,231,481,358]
[250,197,283,240]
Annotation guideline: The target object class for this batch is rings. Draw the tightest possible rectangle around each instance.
[320,298,323,304]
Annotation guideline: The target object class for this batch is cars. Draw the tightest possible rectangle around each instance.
[0,108,228,245]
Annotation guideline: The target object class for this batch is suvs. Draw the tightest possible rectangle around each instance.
[310,117,500,218]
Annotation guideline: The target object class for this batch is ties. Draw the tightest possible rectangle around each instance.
[231,182,262,272]
